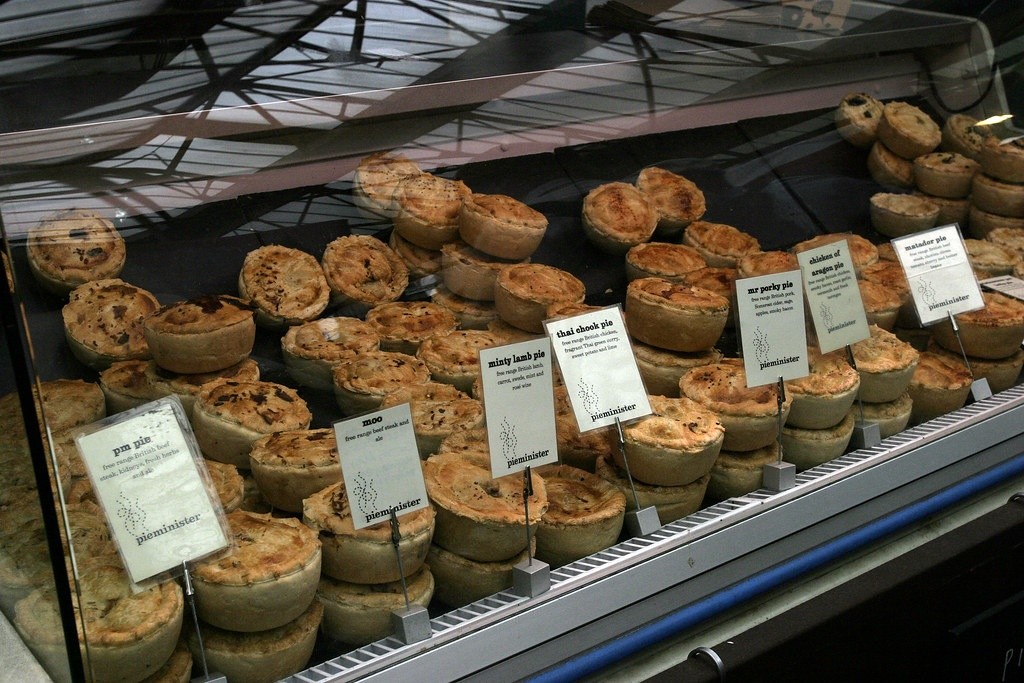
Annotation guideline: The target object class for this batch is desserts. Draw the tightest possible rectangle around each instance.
[0,92,1024,683]
